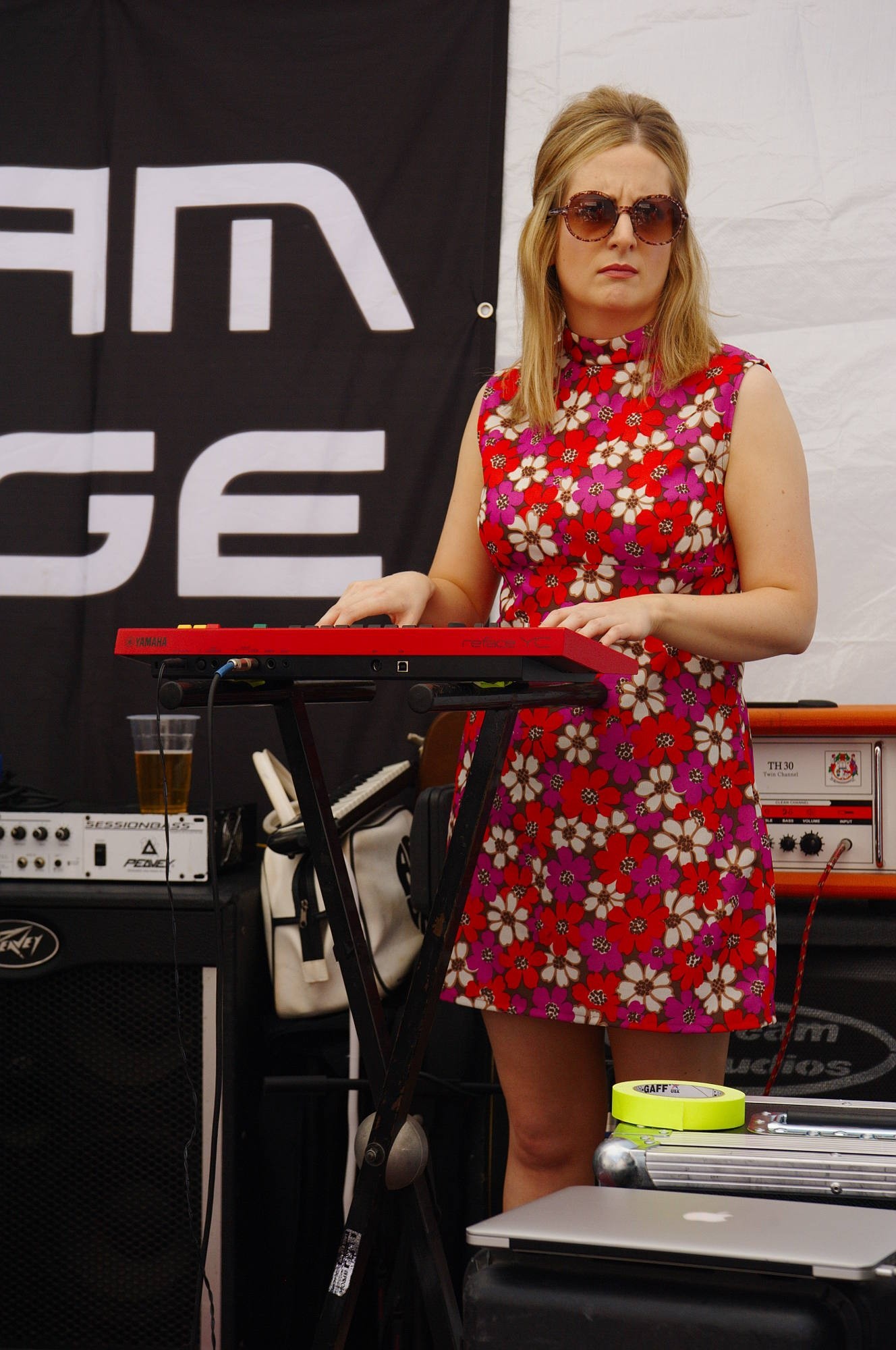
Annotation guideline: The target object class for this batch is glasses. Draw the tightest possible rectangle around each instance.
[548,190,689,246]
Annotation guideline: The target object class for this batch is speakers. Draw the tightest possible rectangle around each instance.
[0,874,342,1350]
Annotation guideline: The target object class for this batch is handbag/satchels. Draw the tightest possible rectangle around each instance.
[251,750,426,1020]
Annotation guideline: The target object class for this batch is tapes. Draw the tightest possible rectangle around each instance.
[610,1078,745,1131]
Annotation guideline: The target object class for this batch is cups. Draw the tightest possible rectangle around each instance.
[127,715,201,815]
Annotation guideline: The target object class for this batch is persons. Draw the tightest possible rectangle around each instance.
[315,89,817,1215]
